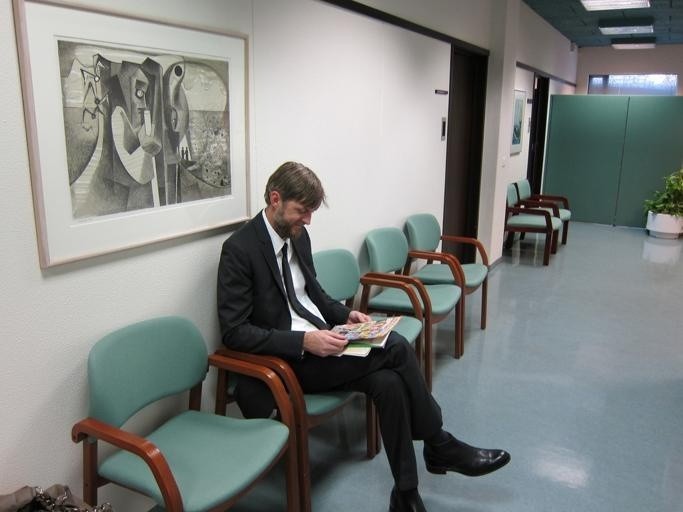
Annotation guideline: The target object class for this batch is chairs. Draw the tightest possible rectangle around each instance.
[358,226,461,392]
[513,179,571,248]
[215,344,377,511]
[505,184,563,265]
[312,249,426,455]
[405,212,491,360]
[71,315,300,512]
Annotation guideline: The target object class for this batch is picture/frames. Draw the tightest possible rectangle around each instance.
[14,1,251,269]
[510,89,527,154]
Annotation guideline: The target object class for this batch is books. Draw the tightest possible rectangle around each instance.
[333,346,373,358]
[327,314,404,351]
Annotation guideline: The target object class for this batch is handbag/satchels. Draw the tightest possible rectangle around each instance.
[0,484,114,511]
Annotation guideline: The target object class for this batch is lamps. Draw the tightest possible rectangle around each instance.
[611,37,657,50]
[598,18,654,35]
[580,0,653,12]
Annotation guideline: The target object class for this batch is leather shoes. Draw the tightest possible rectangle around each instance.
[423,432,510,477]
[390,486,427,512]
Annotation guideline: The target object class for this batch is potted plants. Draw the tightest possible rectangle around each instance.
[642,168,683,239]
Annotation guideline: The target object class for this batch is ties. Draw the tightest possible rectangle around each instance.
[282,242,329,330]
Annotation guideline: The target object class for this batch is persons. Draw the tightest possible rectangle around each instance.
[214,160,511,512]
